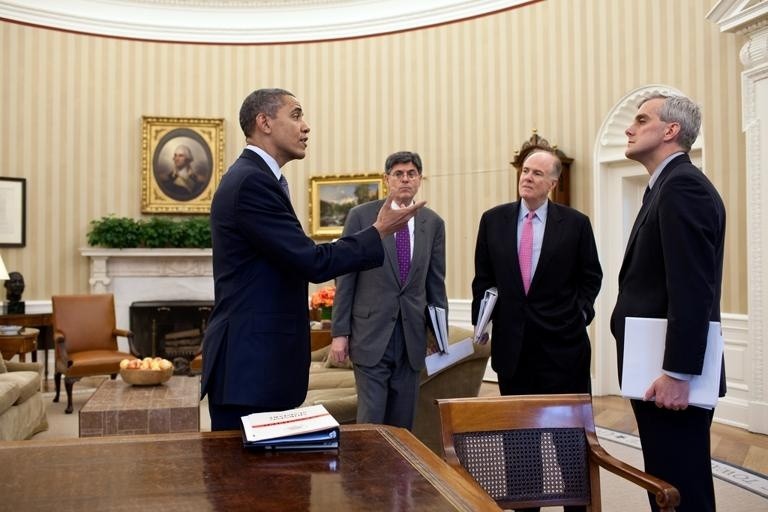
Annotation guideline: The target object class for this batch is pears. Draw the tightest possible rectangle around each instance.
[119,355,173,372]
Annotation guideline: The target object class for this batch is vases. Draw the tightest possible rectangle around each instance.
[320,307,331,319]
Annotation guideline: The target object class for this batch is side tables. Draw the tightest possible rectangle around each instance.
[311,329,331,352]
[0,325,40,362]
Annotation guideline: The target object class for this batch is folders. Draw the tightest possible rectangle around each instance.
[239,404,340,451]
[473,287,499,344]
[425,302,445,352]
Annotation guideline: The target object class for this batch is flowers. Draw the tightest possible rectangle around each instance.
[311,287,336,309]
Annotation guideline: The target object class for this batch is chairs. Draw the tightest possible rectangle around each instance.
[432,393,680,512]
[52,294,143,414]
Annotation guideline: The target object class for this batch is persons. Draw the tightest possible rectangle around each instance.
[197,87,427,430]
[608,89,727,512]
[332,152,449,431]
[161,144,206,195]
[471,146,604,512]
[4,271,25,302]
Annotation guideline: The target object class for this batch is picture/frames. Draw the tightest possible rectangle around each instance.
[141,114,225,213]
[309,172,388,239]
[0,177,26,247]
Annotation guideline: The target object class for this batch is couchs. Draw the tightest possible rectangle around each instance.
[0,351,48,441]
[296,324,492,461]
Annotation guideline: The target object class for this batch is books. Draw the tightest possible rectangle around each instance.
[239,404,341,454]
[475,287,496,343]
[426,301,450,354]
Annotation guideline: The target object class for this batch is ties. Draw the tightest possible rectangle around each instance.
[280,174,291,204]
[395,221,413,290]
[518,209,536,298]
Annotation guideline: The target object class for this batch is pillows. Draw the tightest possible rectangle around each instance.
[324,349,354,369]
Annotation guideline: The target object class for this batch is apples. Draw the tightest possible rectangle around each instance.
[311,286,336,307]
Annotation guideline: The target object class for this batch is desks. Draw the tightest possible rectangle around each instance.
[0,313,54,327]
[0,423,503,512]
